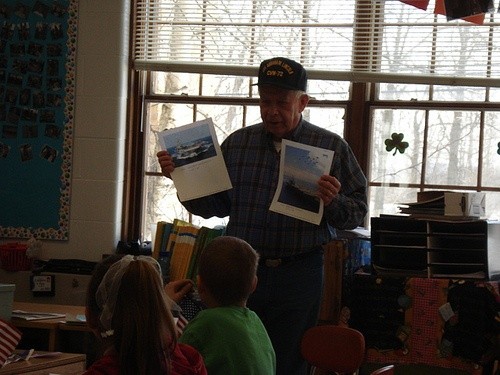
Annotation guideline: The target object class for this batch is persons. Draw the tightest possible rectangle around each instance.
[157,57,368,375]
[84,255,207,375]
[176,236,277,375]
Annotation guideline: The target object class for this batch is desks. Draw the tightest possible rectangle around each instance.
[13,301,94,334]
[0,350,87,375]
[345,266,500,375]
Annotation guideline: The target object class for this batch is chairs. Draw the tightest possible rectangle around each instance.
[301,325,365,375]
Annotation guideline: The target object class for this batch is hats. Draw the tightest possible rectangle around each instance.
[249,58,306,92]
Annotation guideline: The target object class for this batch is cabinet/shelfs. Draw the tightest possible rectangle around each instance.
[370,215,489,278]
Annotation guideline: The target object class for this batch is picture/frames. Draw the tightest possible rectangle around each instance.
[0,0,79,240]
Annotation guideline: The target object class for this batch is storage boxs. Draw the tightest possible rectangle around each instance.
[418,190,468,216]
[0,283,15,320]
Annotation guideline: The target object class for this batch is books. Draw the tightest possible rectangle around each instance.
[11,310,66,321]
[151,217,224,292]
